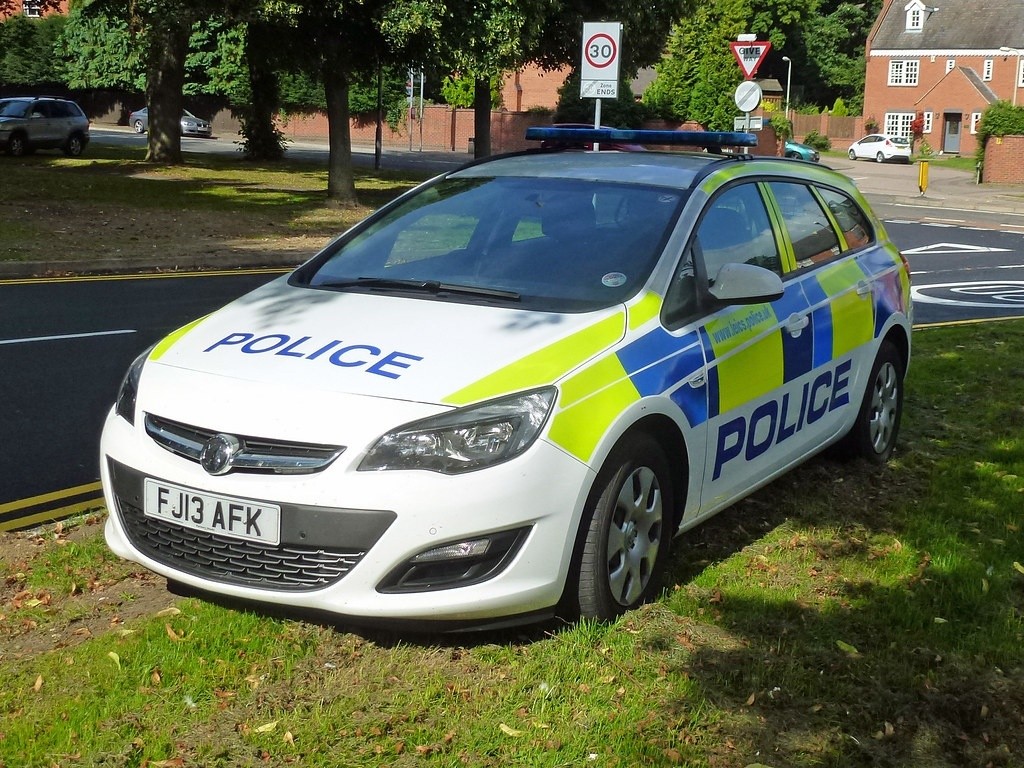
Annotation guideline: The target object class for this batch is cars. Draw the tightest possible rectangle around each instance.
[784,137,820,164]
[129,104,212,137]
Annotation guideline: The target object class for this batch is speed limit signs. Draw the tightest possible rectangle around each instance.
[585,33,617,70]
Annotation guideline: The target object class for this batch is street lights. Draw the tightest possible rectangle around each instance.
[782,56,791,118]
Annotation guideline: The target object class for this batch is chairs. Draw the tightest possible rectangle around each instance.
[696,207,750,279]
[510,193,607,289]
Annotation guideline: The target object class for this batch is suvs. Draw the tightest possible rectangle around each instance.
[847,134,910,163]
[100,122,912,629]
[541,123,649,152]
[0,95,90,156]
